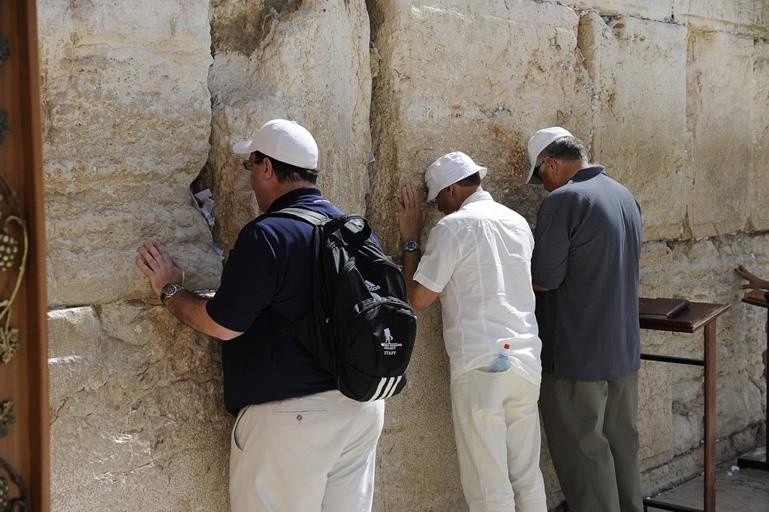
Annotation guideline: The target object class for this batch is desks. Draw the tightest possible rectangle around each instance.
[638,297,731,510]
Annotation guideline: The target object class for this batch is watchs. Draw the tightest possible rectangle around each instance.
[401,240,420,252]
[159,284,182,302]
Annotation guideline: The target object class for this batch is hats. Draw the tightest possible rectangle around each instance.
[525,126,573,185]
[424,150,487,205]
[232,118,318,170]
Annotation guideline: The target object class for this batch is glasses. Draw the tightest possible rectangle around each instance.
[243,158,262,170]
[534,157,554,178]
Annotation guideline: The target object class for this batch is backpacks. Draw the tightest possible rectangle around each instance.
[266,208,417,402]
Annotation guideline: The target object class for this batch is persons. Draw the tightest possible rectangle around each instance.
[526,126,644,512]
[136,119,387,512]
[394,150,547,512]
[734,266,769,304]
